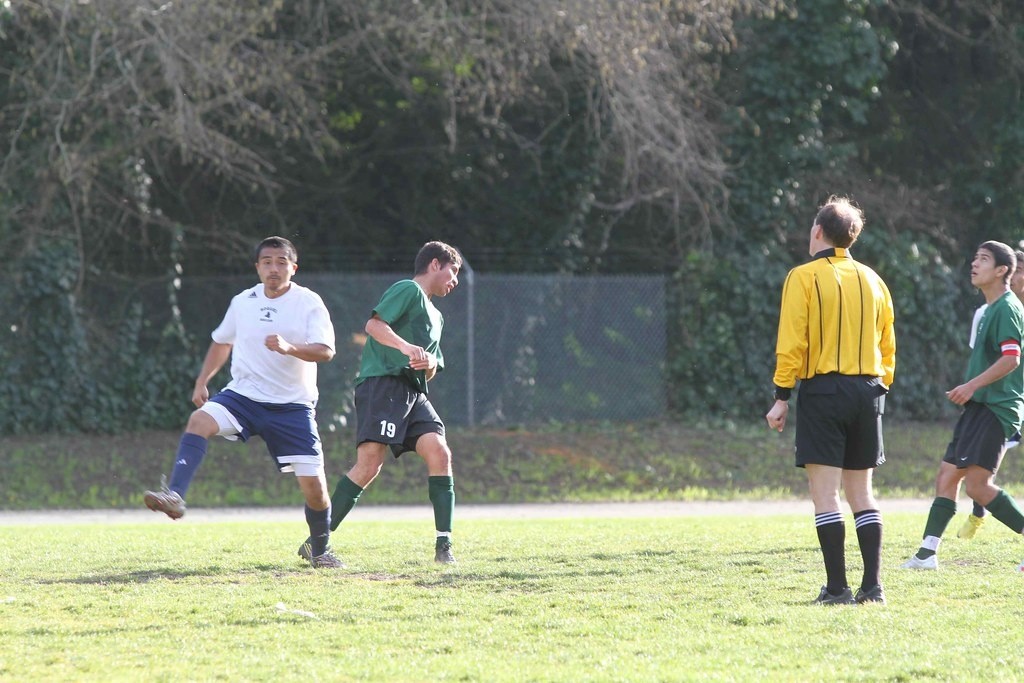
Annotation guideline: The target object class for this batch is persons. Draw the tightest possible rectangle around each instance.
[143,235,340,567]
[955,250,1024,542]
[903,241,1024,572]
[765,200,895,606]
[298,242,463,563]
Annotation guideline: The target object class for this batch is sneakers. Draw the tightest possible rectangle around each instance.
[143,474,185,519]
[309,546,344,571]
[811,586,855,605]
[297,537,312,563]
[853,583,884,604]
[902,554,939,569]
[957,514,984,539]
[434,543,456,567]
